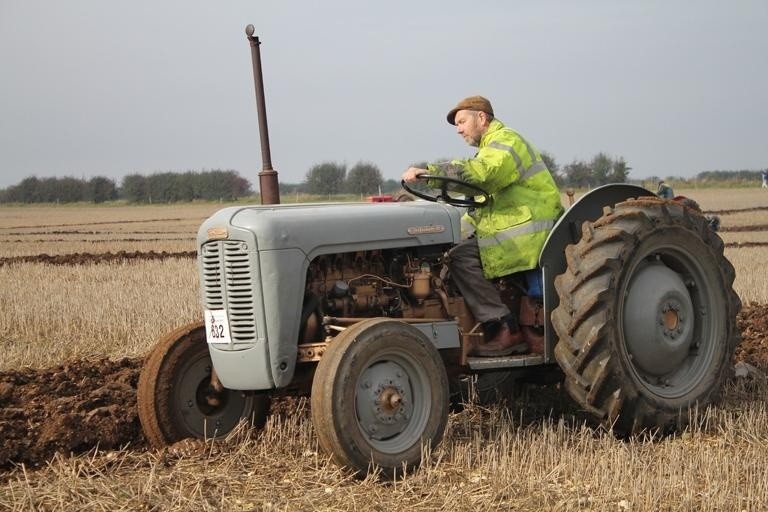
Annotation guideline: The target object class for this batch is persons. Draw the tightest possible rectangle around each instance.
[760,168,768,188]
[656,180,674,199]
[400,96,566,356]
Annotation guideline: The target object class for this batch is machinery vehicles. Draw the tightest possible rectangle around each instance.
[136,24,743,481]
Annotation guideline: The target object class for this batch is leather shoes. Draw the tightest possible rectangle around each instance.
[473,322,530,357]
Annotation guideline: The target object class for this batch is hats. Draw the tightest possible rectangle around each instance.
[446,96,494,126]
